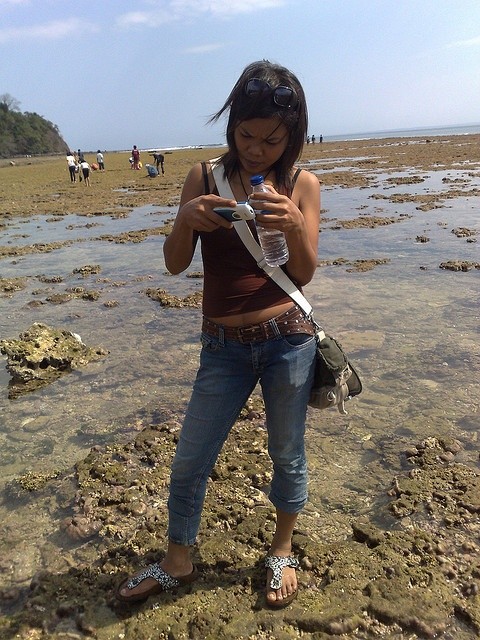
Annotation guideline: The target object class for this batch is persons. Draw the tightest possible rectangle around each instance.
[320,135,322,142]
[74,151,77,161]
[78,149,83,160]
[80,159,91,186]
[129,157,134,169]
[138,160,142,168]
[66,152,75,181]
[307,136,309,145]
[96,150,104,172]
[153,153,165,178]
[76,160,82,182]
[91,163,98,171]
[132,145,140,170]
[145,164,158,177]
[312,135,315,143]
[164,60,321,303]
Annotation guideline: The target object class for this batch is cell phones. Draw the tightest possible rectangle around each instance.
[213,201,257,222]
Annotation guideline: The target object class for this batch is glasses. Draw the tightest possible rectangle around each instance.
[245,79,299,108]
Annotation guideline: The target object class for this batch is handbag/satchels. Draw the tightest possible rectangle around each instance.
[209,156,362,415]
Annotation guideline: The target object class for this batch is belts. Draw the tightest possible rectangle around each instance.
[202,305,314,346]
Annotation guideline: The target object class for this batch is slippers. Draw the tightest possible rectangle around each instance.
[116,562,198,602]
[265,555,299,607]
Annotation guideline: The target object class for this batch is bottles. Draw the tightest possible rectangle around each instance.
[250,174,290,267]
